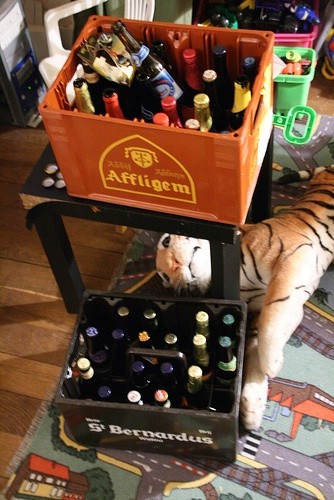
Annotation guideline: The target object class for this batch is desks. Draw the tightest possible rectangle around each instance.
[20,127,278,314]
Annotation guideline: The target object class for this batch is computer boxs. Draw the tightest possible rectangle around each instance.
[0,0,43,128]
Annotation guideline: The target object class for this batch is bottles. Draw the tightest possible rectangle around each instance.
[151,40,170,63]
[193,93,217,133]
[212,45,233,108]
[202,69,224,133]
[111,20,188,108]
[77,306,237,408]
[153,112,169,127]
[226,75,251,132]
[242,56,258,94]
[73,61,128,120]
[183,47,204,118]
[136,71,162,124]
[161,95,184,129]
[185,118,201,131]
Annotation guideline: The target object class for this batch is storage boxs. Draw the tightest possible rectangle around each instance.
[272,19,319,119]
[56,289,247,463]
[39,16,273,225]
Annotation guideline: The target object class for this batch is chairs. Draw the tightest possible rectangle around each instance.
[38,0,155,100]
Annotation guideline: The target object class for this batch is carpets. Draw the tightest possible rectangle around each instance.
[0,111,334,500]
